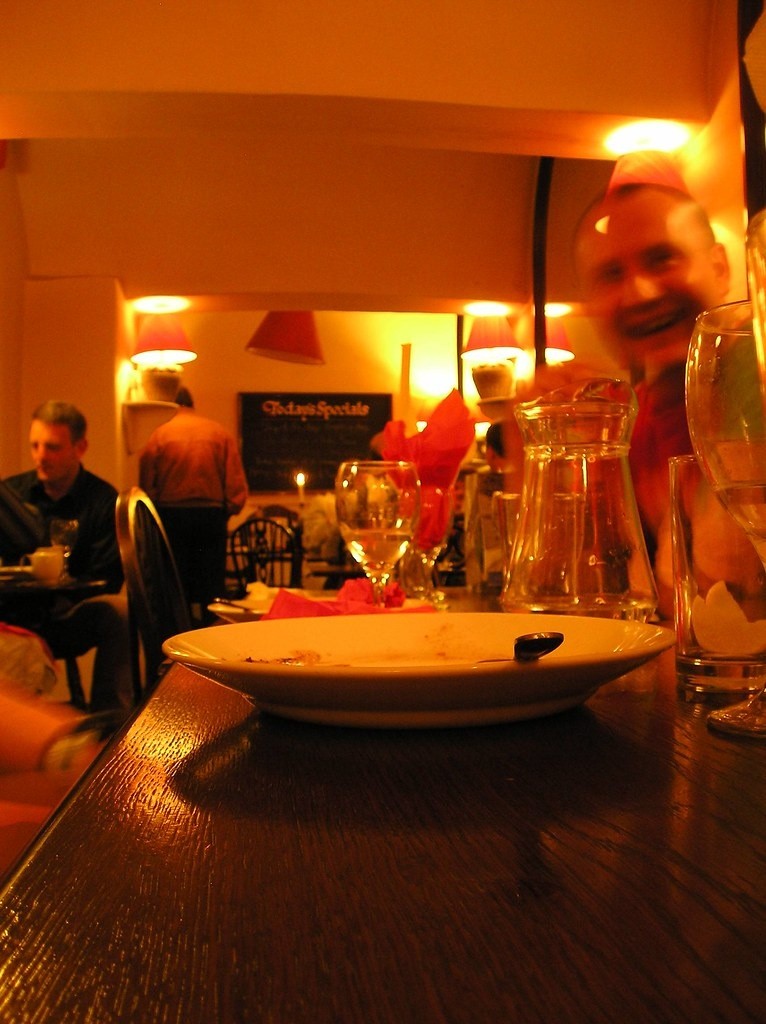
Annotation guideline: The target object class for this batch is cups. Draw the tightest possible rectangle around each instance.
[666,454,766,693]
[30,551,64,587]
[741,213,766,405]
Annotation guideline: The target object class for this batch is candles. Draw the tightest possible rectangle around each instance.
[297,473,305,505]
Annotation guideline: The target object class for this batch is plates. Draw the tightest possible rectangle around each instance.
[159,611,678,729]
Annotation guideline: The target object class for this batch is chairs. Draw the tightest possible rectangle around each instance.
[116,487,188,702]
[231,505,305,592]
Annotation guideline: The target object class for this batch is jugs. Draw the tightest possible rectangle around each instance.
[496,375,660,623]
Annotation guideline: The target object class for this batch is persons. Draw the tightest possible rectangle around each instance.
[139,386,248,607]
[479,422,506,495]
[0,399,137,714]
[571,184,732,538]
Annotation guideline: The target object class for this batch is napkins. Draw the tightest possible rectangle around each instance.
[371,389,477,552]
[258,587,438,620]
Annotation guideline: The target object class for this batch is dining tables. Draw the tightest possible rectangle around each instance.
[0,597,766,1024]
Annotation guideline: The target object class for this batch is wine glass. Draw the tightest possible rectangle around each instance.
[333,458,420,609]
[683,299,766,740]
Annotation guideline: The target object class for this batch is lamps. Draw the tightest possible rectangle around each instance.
[521,317,574,364]
[459,316,523,363]
[131,317,198,366]
[247,310,325,366]
